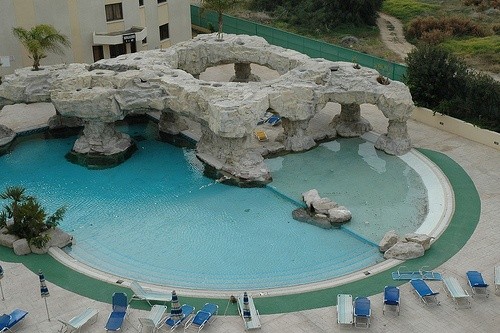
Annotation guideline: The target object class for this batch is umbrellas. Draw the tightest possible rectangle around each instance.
[0,265,5,301]
[242,292,252,328]
[38,271,50,323]
[171,290,184,326]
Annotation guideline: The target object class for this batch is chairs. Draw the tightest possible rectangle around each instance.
[336,294,354,329]
[256,129,270,142]
[157,303,196,333]
[103,292,131,333]
[57,306,101,333]
[492,265,500,296]
[465,270,490,299]
[382,285,401,316]
[237,295,262,331]
[263,114,281,129]
[0,308,29,333]
[352,296,372,329]
[408,278,441,307]
[128,279,172,307]
[441,276,473,309]
[184,303,220,333]
[137,304,168,333]
